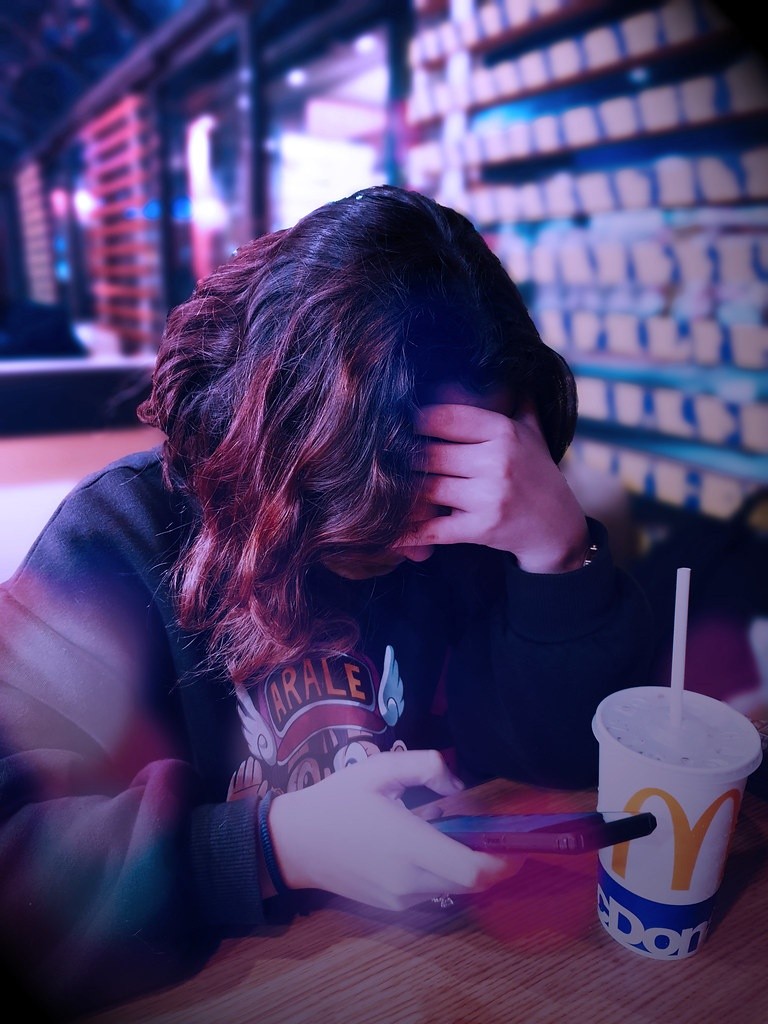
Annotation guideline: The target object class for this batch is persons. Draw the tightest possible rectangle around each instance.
[1,184,629,1022]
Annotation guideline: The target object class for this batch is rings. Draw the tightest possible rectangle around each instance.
[430,893,455,908]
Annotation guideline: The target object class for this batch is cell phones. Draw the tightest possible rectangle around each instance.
[422,815,658,856]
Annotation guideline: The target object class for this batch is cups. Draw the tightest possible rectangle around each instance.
[592,685,762,960]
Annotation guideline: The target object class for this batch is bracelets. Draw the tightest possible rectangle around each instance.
[583,540,599,565]
